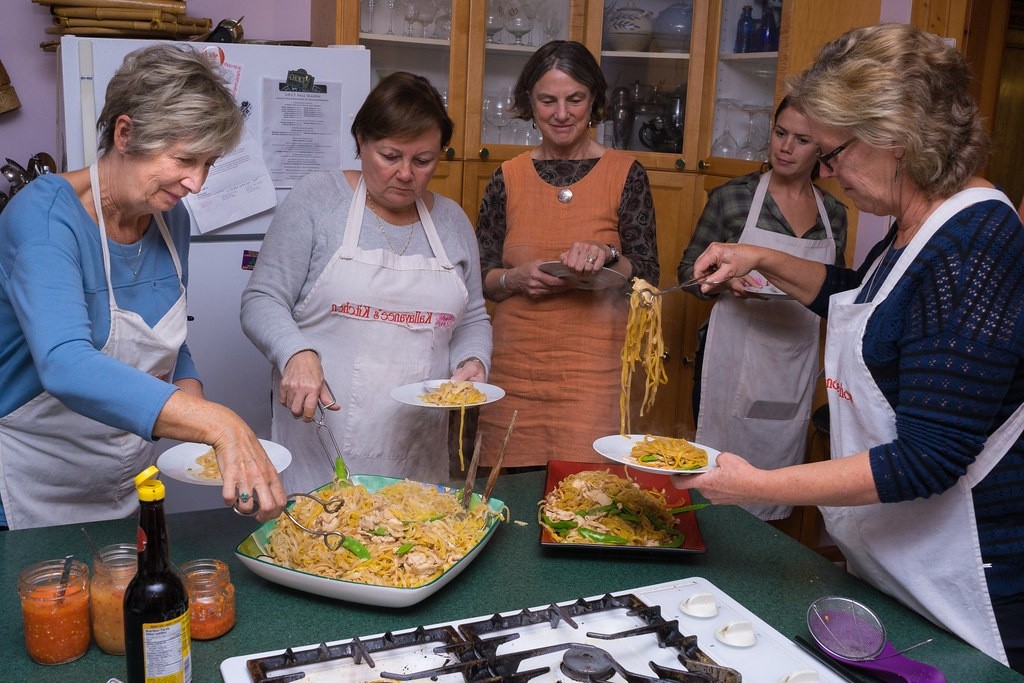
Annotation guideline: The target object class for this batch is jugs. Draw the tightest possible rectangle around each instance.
[639,114,683,154]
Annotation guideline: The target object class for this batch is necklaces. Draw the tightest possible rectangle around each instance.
[543,136,592,203]
[366,188,416,254]
[98,202,145,275]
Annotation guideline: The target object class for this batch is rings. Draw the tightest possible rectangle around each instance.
[237,492,253,502]
[588,258,595,264]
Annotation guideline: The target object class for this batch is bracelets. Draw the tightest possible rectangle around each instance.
[456,358,486,380]
[500,268,515,293]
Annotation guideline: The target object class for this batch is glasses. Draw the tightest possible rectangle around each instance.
[814,137,858,171]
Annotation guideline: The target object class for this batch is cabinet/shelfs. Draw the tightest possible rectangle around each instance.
[585,0,878,554]
[310,0,586,229]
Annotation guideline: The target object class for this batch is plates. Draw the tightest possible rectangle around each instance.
[539,461,708,555]
[592,434,721,475]
[234,474,506,609]
[155,439,293,487]
[743,286,796,300]
[389,380,506,411]
[539,261,628,291]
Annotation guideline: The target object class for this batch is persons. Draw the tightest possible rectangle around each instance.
[240,73,493,500]
[0,44,287,522]
[475,41,660,475]
[678,93,848,523]
[670,23,1024,674]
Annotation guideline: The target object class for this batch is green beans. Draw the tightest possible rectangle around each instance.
[336,457,448,558]
[638,454,703,471]
[540,499,711,548]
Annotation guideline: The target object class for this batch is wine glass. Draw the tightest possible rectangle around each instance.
[363,0,562,145]
[712,98,773,163]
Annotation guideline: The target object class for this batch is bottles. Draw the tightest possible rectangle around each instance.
[121,463,194,683]
[178,559,236,640]
[732,4,778,53]
[91,543,137,655]
[18,558,89,666]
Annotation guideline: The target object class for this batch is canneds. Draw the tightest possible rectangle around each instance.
[20,544,235,666]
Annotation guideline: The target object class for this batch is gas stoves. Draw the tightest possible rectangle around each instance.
[219,575,855,683]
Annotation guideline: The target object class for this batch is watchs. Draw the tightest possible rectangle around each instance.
[603,243,620,268]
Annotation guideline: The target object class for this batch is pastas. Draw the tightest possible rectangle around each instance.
[632,433,708,471]
[196,445,222,480]
[619,277,668,439]
[417,380,486,471]
[263,473,510,588]
[538,464,673,547]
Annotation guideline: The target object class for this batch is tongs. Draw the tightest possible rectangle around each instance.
[626,270,713,307]
[232,483,346,552]
[289,378,351,485]
